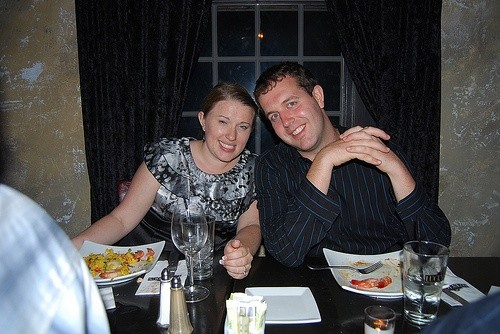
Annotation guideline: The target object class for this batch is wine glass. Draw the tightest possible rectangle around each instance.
[171,203,210,302]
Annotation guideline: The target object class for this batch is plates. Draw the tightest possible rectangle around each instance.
[79,240,165,285]
[322,247,422,298]
[244,287,321,324]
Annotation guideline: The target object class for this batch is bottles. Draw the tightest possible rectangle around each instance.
[165,275,194,334]
[155,268,171,328]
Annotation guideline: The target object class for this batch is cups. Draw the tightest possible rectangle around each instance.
[403,240,450,329]
[180,215,216,280]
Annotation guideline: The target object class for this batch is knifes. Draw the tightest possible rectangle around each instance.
[442,288,469,304]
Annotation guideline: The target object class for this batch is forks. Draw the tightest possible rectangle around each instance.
[306,261,382,274]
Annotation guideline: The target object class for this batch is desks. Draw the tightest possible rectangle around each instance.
[101,254,500,334]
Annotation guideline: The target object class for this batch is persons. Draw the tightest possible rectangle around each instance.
[254,63,451,269]
[70,82,260,280]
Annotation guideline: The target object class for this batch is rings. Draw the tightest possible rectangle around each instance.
[244,272,246,277]
[244,265,247,271]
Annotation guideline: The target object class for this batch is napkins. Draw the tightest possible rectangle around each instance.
[427,257,486,308]
[135,259,189,296]
